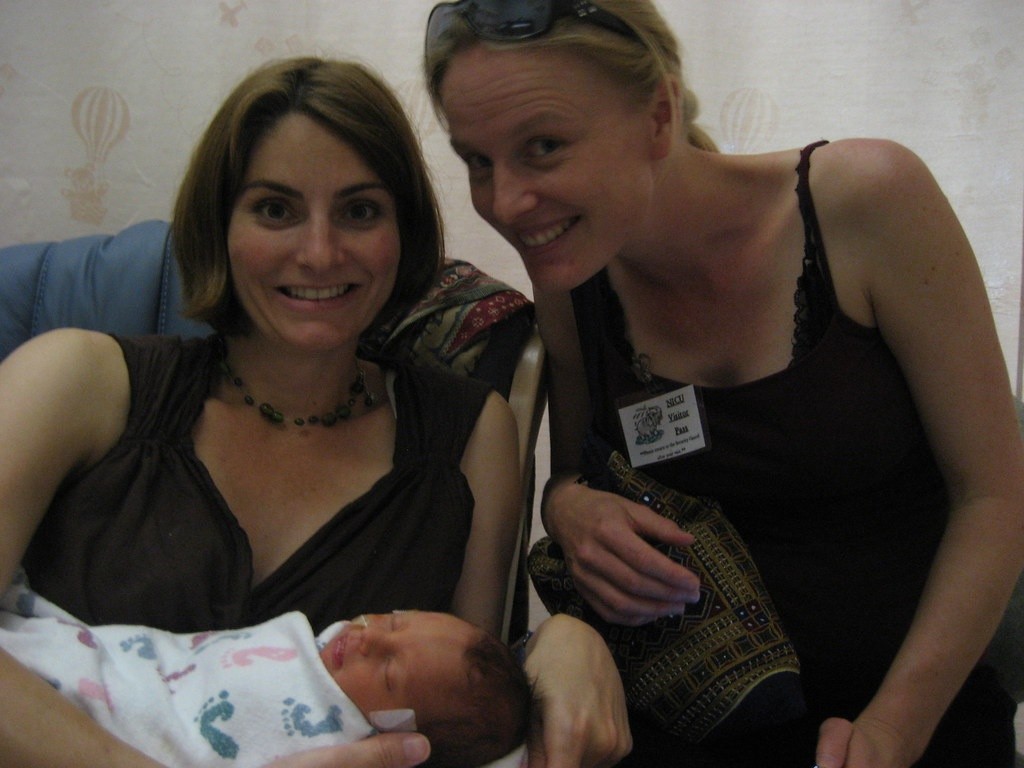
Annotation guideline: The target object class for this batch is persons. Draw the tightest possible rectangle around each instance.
[423,0,1024,768]
[0,59,635,768]
[1,578,540,768]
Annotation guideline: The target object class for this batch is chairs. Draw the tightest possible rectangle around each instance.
[0,218,548,768]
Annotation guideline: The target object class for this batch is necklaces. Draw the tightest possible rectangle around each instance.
[219,358,372,427]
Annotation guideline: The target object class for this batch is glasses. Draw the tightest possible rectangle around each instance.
[424,0,647,60]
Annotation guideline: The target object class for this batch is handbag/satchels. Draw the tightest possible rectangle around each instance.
[525,451,802,742]
